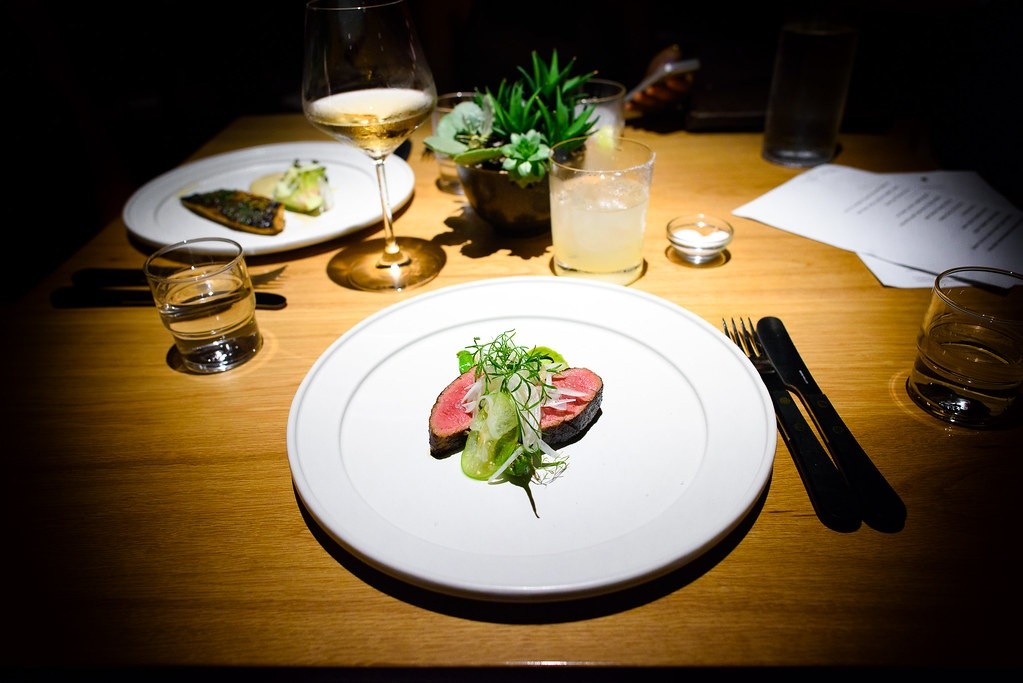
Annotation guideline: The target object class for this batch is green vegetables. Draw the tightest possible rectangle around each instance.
[456,329,570,479]
[274,158,327,197]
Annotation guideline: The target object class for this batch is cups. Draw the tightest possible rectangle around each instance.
[761,23,855,167]
[143,238,264,374]
[548,134,655,281]
[428,89,491,191]
[560,80,623,146]
[907,267,1023,429]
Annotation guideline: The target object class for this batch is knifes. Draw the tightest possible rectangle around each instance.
[756,316,908,531]
[50,287,303,305]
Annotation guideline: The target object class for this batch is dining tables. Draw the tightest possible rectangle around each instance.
[2,106,1023,681]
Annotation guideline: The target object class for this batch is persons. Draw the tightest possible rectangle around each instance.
[413,0,697,123]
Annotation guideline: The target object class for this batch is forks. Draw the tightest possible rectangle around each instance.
[720,313,863,534]
[70,265,286,287]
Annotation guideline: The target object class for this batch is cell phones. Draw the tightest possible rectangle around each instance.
[622,58,701,105]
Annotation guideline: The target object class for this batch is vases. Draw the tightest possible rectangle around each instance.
[453,164,603,235]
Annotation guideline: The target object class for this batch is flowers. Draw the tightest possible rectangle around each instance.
[454,50,606,185]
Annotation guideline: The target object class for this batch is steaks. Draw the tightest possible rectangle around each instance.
[429,365,604,456]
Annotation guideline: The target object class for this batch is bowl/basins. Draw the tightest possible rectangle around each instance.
[667,215,734,265]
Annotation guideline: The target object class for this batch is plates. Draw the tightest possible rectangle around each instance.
[122,139,414,258]
[283,274,777,602]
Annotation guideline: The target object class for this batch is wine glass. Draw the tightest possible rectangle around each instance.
[302,0,442,292]
